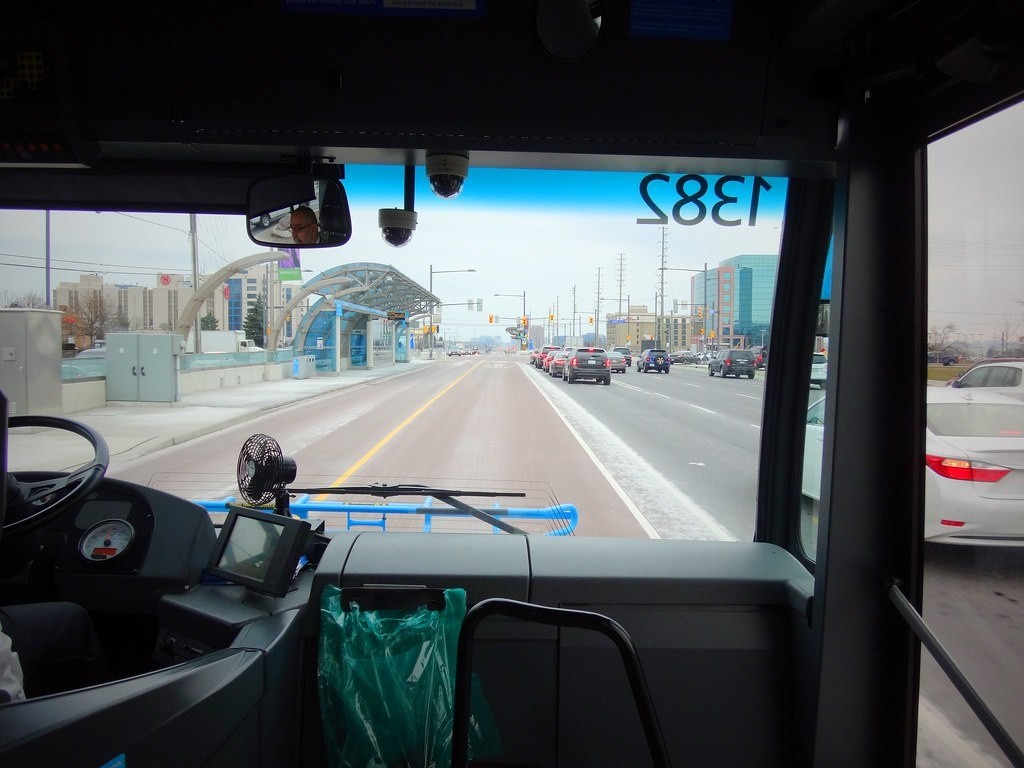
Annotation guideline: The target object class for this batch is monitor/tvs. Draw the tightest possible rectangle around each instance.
[207,505,313,598]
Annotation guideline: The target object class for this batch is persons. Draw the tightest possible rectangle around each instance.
[290,206,321,245]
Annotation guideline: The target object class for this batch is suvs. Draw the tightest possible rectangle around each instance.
[637,349,671,373]
[608,346,632,367]
[707,348,756,379]
[562,346,612,385]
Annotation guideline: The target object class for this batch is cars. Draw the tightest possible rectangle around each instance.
[435,339,495,356]
[693,350,718,364]
[749,345,768,370]
[529,346,574,378]
[810,352,828,389]
[801,382,1024,548]
[942,354,1024,386]
[669,351,701,365]
[605,352,626,373]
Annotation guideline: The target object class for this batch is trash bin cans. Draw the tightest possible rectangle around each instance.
[395,347,406,363]
[292,355,316,380]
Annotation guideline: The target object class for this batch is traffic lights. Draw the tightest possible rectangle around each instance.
[589,316,593,324]
[489,314,494,324]
[699,328,703,335]
[698,307,703,317]
[550,314,554,320]
[522,316,528,325]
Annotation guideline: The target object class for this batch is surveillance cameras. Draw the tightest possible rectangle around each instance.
[378,207,419,247]
[425,154,469,199]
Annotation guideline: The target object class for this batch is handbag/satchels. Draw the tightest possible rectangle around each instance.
[315,582,504,768]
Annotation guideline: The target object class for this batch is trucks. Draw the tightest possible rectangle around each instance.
[185,328,264,353]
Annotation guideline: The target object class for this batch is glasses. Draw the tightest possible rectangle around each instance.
[290,223,314,233]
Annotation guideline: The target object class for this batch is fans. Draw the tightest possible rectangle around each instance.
[237,434,298,517]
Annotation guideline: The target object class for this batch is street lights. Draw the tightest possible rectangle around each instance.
[443,328,450,349]
[600,295,630,347]
[657,263,707,345]
[561,316,581,346]
[759,330,767,346]
[494,290,525,336]
[574,309,597,348]
[429,265,477,359]
